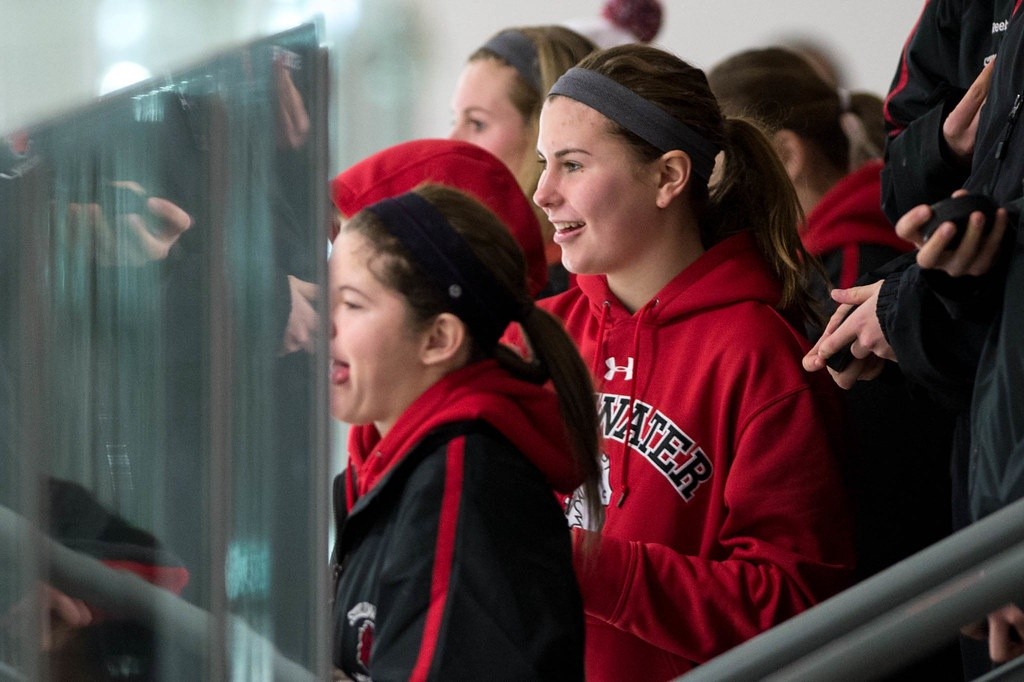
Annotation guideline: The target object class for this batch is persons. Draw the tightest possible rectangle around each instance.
[0,476,188,654]
[321,0,1024,682]
